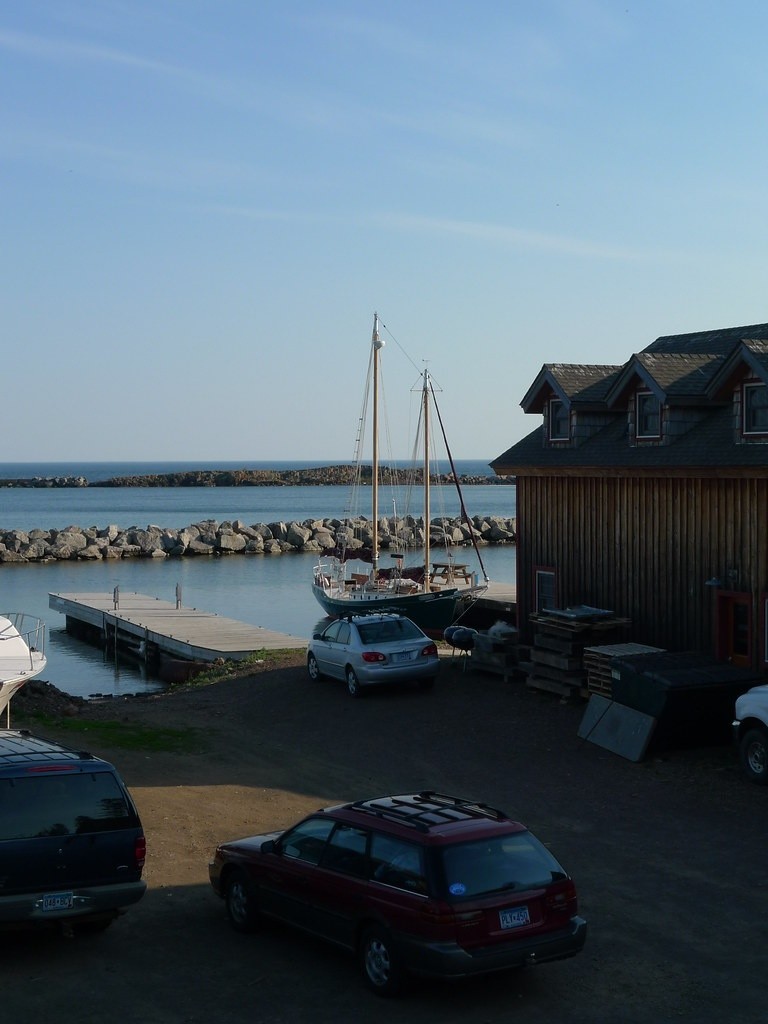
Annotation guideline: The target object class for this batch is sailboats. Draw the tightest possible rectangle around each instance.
[311,308,490,630]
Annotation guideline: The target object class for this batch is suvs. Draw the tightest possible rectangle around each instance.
[0,728,148,944]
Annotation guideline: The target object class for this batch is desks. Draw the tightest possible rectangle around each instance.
[430,563,470,586]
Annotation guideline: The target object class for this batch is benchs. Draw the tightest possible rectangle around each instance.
[430,573,470,578]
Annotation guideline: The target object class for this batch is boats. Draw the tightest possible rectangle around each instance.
[0,611,48,719]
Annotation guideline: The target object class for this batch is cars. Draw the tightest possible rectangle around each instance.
[730,683,768,788]
[205,788,588,996]
[306,606,442,698]
